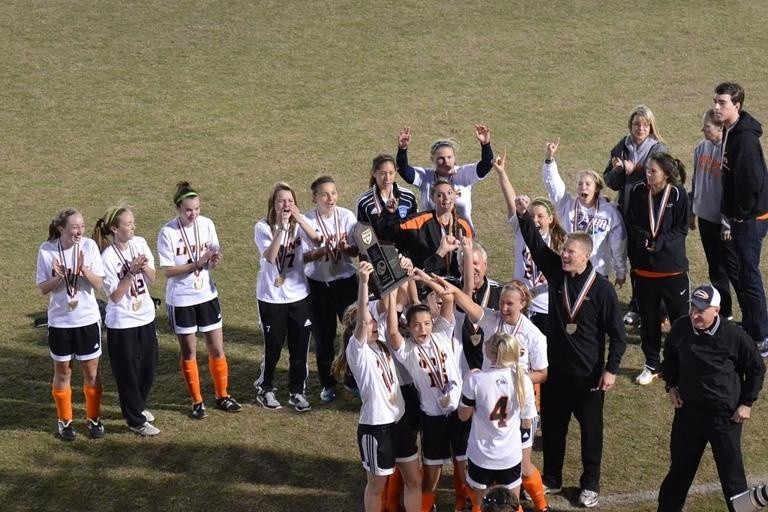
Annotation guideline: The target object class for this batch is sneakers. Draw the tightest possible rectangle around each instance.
[128,410,160,435]
[58,419,76,441]
[215,395,242,412]
[289,393,311,412]
[622,310,639,325]
[524,480,562,500]
[192,402,208,418]
[256,389,282,410]
[579,490,599,508]
[87,417,104,438]
[637,367,662,386]
[320,385,335,401]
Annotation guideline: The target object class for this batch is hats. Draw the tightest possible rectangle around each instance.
[687,285,721,310]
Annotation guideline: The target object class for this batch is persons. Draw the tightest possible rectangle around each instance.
[686,108,750,332]
[35,207,107,442]
[657,284,765,510]
[156,182,246,419]
[606,104,668,325]
[624,154,690,386]
[92,206,163,436]
[252,123,628,512]
[712,81,768,357]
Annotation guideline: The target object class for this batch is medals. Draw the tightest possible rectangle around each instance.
[192,279,203,290]
[132,298,142,311]
[646,241,656,251]
[68,300,79,312]
